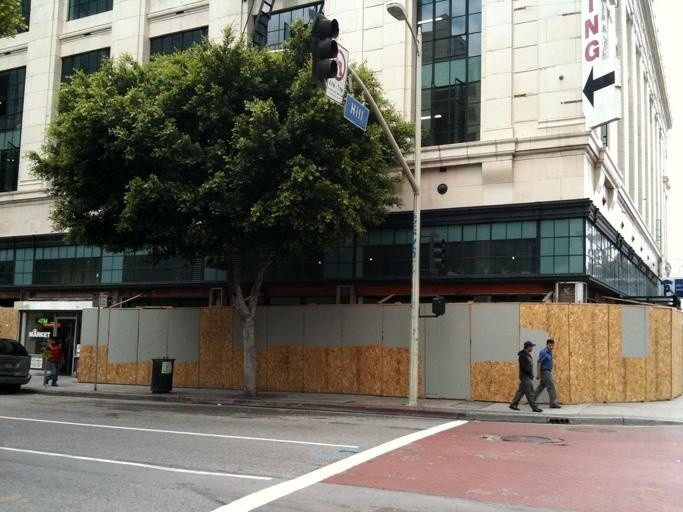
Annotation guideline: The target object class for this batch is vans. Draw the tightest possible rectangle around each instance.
[0,337,32,392]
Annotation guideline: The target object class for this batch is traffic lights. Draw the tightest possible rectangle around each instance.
[310,11,341,91]
[432,297,445,315]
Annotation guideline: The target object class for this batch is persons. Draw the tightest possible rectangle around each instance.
[44,336,64,386]
[509,341,543,412]
[534,339,562,409]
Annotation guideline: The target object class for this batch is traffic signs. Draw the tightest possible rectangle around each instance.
[344,93,369,133]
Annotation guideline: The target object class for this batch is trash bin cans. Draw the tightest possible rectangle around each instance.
[148,358,175,394]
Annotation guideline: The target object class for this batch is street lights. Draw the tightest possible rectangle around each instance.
[380,0,424,404]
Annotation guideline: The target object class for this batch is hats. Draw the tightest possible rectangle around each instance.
[524,341,536,349]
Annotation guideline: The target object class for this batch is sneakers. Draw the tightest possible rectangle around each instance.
[533,408,543,413]
[509,405,520,410]
[550,404,562,409]
